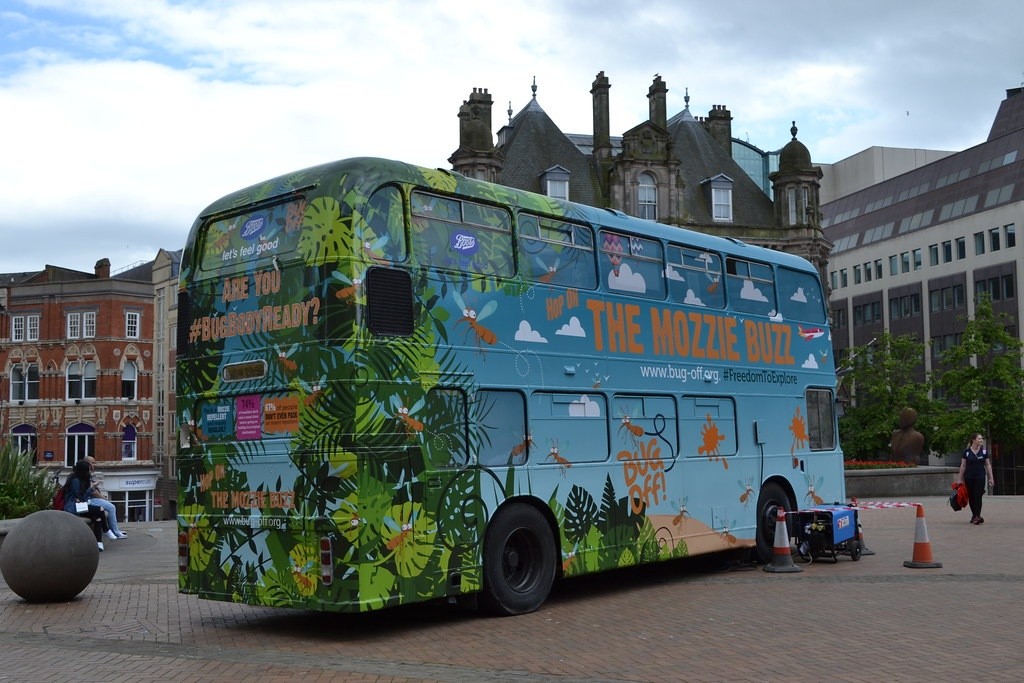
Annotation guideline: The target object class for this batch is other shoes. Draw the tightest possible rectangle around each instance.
[974,517,983,524]
[103,529,117,540]
[116,531,128,539]
[970,516,975,523]
[98,542,104,551]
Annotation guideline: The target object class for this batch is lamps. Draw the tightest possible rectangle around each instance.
[19,401,24,405]
[75,400,80,404]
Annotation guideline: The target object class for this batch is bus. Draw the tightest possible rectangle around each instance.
[176,157,856,617]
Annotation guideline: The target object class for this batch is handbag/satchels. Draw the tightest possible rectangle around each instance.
[946,489,961,512]
[76,502,89,514]
[951,483,969,508]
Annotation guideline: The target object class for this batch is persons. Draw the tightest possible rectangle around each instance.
[958,431,995,524]
[84,456,128,539]
[65,460,118,551]
[890,406,925,464]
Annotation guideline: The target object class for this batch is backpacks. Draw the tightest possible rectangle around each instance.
[52,475,75,509]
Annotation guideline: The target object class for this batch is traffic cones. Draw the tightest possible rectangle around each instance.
[840,498,876,557]
[763,507,804,572]
[903,504,942,568]
